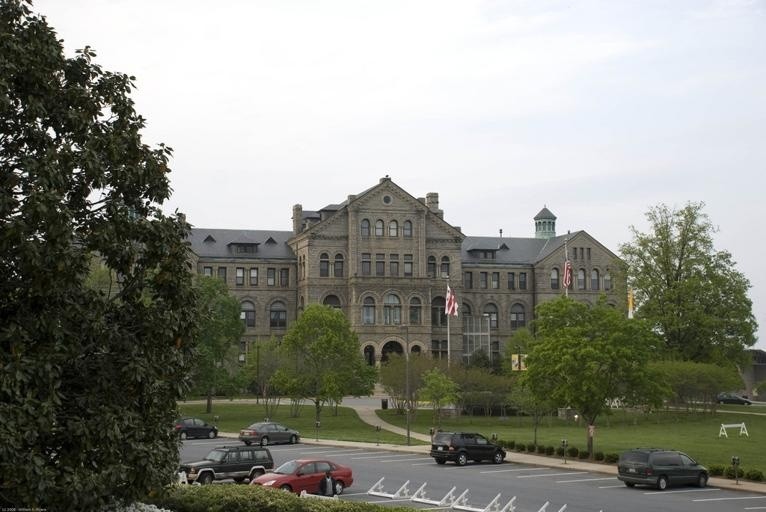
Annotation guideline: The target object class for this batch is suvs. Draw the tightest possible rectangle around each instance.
[179,445,274,486]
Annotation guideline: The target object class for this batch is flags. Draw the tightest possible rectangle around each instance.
[445,285,459,317]
[562,259,572,289]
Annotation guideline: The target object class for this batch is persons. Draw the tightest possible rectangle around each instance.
[319,470,336,497]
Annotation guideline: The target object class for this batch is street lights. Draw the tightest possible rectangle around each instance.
[397,324,411,444]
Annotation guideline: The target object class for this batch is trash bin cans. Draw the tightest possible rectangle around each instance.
[382,399,388,409]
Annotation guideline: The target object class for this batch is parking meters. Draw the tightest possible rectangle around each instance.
[561,438,569,464]
[430,428,435,443]
[491,432,497,441]
[375,424,381,446]
[314,421,321,441]
[212,415,219,427]
[263,417,269,422]
[731,454,740,485]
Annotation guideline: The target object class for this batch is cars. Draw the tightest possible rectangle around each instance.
[249,458,353,495]
[716,392,753,406]
[429,431,506,466]
[617,447,709,489]
[239,421,300,446]
[171,417,218,440]
